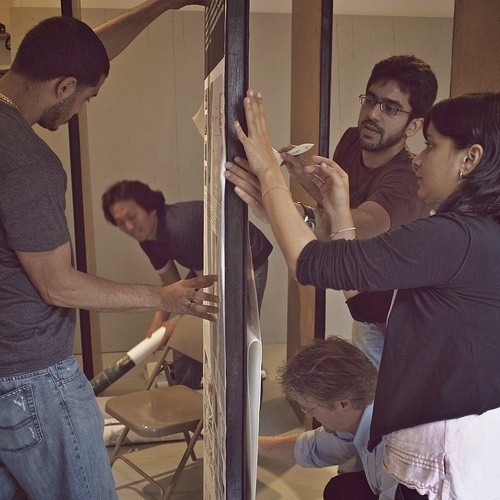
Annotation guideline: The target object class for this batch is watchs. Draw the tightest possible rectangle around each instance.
[296,201,317,232]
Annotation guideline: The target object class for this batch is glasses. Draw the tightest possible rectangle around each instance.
[299,404,321,417]
[359,94,411,116]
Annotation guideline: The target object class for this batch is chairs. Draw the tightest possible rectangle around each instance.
[105,314,203,500]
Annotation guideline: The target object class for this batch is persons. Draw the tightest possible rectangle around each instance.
[1,16,125,500]
[223,88,499,500]
[101,179,274,391]
[225,54,439,373]
[258,332,431,500]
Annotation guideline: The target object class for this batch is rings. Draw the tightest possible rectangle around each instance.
[187,301,192,308]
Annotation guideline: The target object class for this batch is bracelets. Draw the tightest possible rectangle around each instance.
[261,185,290,201]
[328,227,355,239]
